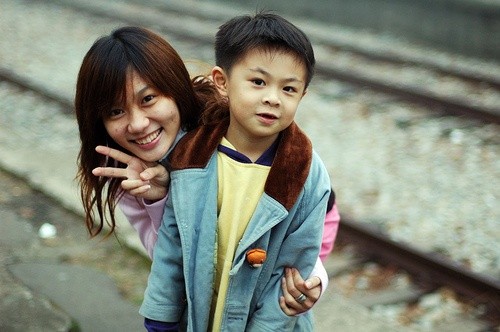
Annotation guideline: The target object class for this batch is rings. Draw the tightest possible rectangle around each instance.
[295,294,307,304]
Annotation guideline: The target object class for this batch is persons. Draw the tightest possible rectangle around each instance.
[73,26,341,316]
[139,11,331,331]
